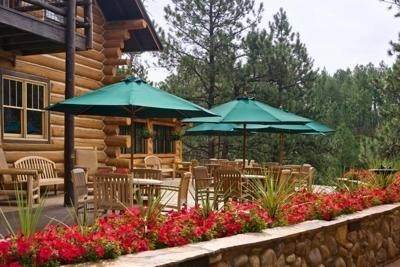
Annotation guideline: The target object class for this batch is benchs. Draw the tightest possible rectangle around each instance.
[14,157,64,196]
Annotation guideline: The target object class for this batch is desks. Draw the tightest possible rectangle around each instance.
[104,178,161,215]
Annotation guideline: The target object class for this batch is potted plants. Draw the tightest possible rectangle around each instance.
[136,128,156,140]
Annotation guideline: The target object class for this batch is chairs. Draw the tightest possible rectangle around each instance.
[142,146,318,205]
[0,168,41,207]
[152,172,190,214]
[93,172,134,224]
[129,169,162,205]
[71,169,111,227]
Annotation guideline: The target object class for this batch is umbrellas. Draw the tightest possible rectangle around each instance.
[181,94,337,167]
[41,75,221,171]
